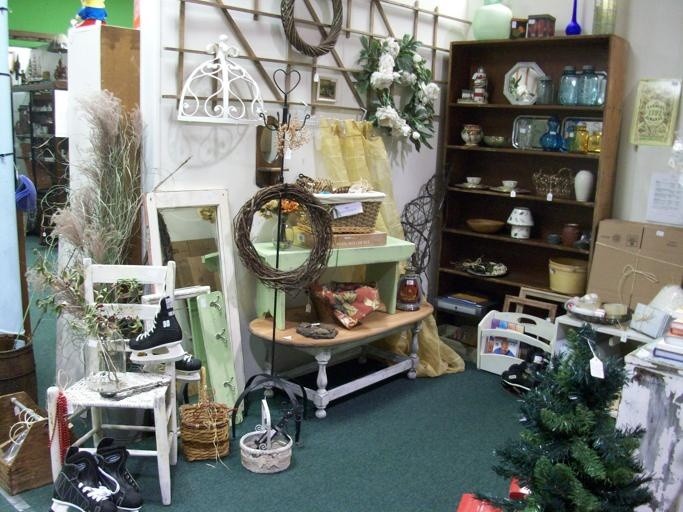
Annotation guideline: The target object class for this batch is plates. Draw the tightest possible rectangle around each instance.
[454,183,491,189]
[564,302,635,324]
[490,185,531,193]
[465,261,508,277]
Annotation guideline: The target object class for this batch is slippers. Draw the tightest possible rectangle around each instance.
[16,174,37,212]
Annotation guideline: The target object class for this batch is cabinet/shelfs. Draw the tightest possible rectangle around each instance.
[553,317,683,512]
[426,33,629,364]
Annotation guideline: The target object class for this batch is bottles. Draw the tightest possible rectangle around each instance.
[518,65,602,153]
[250,411,292,449]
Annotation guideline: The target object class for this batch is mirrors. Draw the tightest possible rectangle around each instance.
[146,188,246,435]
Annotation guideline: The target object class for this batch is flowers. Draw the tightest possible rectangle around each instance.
[359,36,442,152]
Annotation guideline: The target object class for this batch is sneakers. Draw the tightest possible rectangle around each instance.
[129,296,182,350]
[53,446,120,512]
[502,347,550,392]
[97,438,143,508]
[175,353,201,371]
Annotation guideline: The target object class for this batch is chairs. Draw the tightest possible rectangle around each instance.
[46,257,177,498]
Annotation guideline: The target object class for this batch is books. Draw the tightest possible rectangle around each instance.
[654,344,683,363]
[669,322,683,336]
[662,332,683,348]
[486,317,525,358]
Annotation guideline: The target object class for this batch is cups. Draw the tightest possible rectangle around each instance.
[466,176,482,185]
[511,225,531,239]
[547,232,560,245]
[502,180,518,189]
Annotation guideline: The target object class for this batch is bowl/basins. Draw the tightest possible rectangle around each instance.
[466,218,505,233]
[507,207,535,227]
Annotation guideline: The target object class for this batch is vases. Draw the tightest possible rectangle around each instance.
[574,170,594,203]
[565,0,581,34]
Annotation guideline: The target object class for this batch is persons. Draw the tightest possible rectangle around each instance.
[494,340,516,357]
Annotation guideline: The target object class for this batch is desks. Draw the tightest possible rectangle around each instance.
[247,302,434,419]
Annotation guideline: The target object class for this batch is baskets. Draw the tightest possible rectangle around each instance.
[297,186,386,233]
[239,399,293,474]
[310,281,376,323]
[531,168,574,199]
[179,366,229,462]
